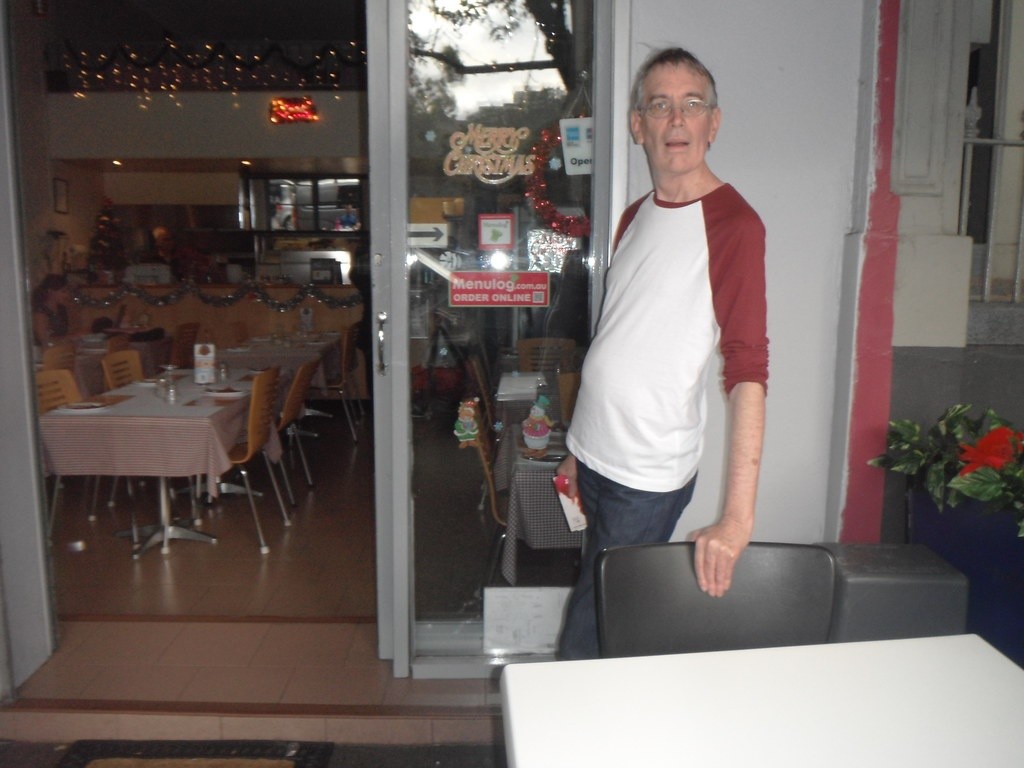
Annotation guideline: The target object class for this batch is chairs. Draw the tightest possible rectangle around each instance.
[30,322,364,563]
[458,326,585,588]
[594,537,842,660]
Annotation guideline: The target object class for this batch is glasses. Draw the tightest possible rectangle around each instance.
[636,98,715,120]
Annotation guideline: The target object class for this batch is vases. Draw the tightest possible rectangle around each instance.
[903,488,1024,668]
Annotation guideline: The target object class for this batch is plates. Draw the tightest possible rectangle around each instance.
[305,339,331,344]
[133,379,165,387]
[81,333,105,342]
[225,345,251,352]
[55,403,108,413]
[322,330,343,336]
[205,389,249,398]
[250,335,274,342]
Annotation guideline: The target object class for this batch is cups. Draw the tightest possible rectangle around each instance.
[214,362,233,387]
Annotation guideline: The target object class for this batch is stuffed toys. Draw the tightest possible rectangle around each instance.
[522,395,554,458]
[454,397,482,448]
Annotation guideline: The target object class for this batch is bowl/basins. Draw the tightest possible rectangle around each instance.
[522,428,551,449]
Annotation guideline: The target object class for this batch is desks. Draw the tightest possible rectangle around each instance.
[497,631,1024,768]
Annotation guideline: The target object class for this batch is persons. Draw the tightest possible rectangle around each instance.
[144,227,183,279]
[349,237,373,398]
[33,274,84,350]
[555,48,772,660]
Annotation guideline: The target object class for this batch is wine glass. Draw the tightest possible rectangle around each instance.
[159,363,182,405]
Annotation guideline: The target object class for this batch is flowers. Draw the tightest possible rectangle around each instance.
[867,401,1024,515]
